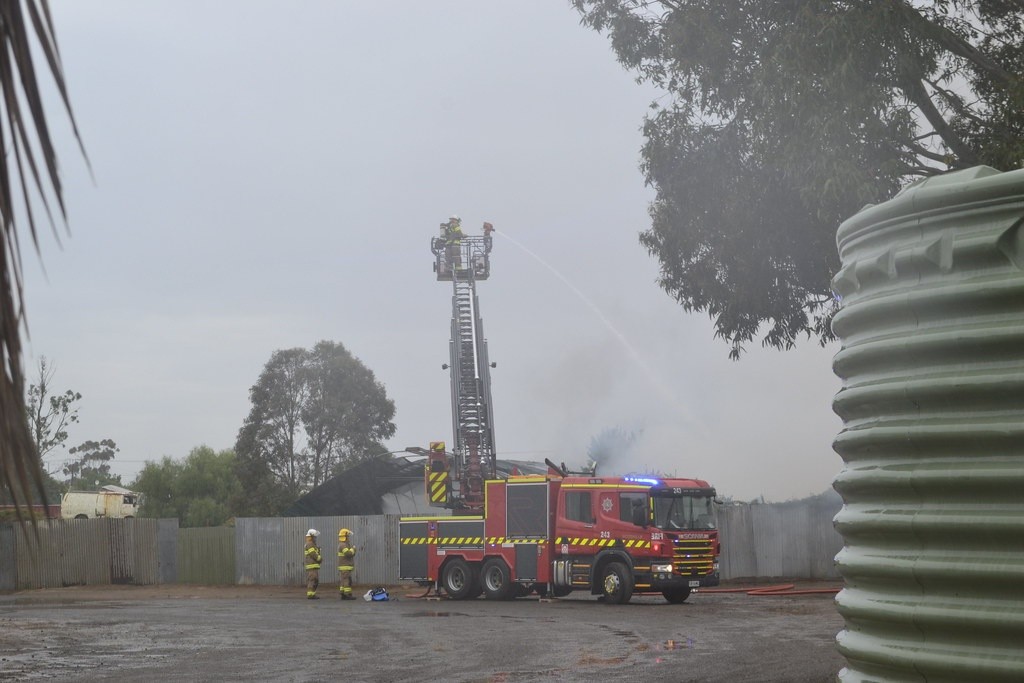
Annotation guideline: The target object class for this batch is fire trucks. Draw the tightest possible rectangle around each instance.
[393,224,725,604]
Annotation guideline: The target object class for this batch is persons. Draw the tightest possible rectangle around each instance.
[304,529,321,600]
[444,215,463,273]
[337,529,356,600]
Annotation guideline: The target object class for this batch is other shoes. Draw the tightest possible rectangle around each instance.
[308,595,319,599]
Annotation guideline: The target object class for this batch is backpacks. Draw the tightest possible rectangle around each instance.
[364,587,390,602]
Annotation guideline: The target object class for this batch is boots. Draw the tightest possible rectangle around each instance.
[341,590,356,600]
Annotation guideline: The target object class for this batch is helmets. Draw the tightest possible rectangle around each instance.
[306,528,320,537]
[338,528,350,541]
